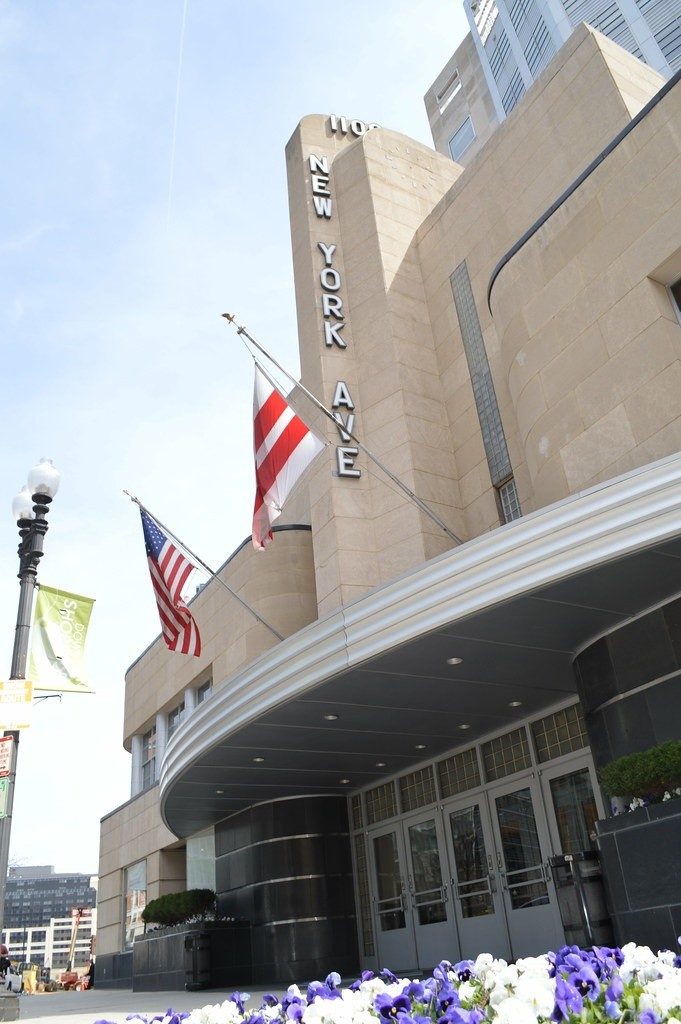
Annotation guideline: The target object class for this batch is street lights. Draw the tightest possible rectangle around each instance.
[0,458,62,957]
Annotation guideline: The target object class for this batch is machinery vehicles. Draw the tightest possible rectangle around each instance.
[59,903,92,992]
[36,966,58,992]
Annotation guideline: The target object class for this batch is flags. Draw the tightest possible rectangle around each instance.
[29,585,96,693]
[252,362,325,552]
[139,509,203,657]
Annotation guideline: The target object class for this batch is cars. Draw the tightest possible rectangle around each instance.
[5,962,47,992]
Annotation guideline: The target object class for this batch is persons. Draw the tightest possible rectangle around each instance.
[84,959,95,990]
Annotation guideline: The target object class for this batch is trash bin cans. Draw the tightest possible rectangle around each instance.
[184,931,215,991]
[547,850,616,952]
